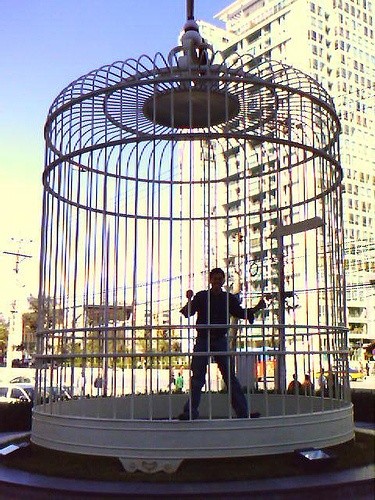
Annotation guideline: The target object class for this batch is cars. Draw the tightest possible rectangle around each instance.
[0,376,79,403]
[315,364,365,381]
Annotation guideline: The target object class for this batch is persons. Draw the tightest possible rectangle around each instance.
[79,371,86,396]
[167,365,184,394]
[359,361,363,379]
[366,360,370,376]
[94,373,103,395]
[288,366,341,396]
[179,268,267,420]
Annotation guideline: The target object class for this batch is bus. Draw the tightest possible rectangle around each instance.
[236,347,279,381]
